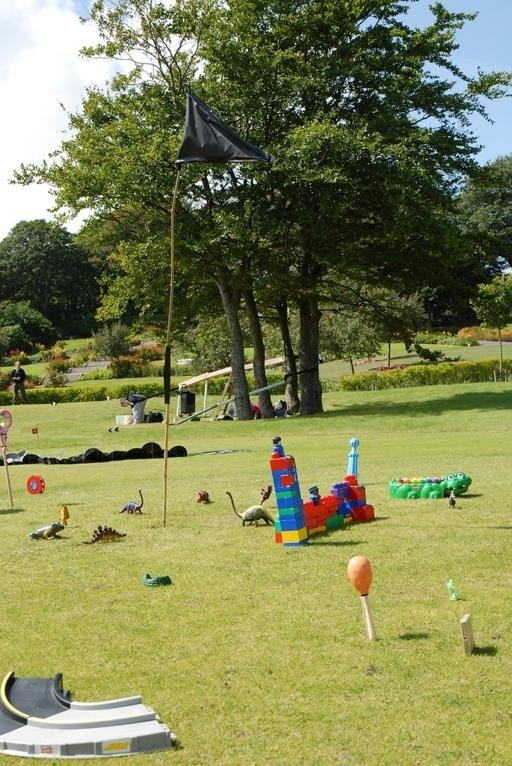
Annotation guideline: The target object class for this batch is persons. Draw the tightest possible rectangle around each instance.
[120,394,147,427]
[11,361,30,407]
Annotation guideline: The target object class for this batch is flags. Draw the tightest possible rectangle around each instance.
[174,92,274,171]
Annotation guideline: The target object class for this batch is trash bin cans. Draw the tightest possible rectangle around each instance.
[180,389,197,417]
